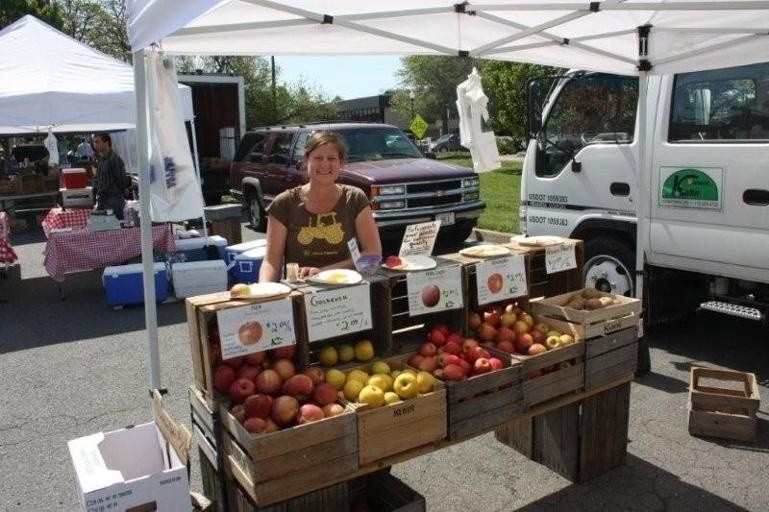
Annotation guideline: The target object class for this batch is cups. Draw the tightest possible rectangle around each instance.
[285,262,299,283]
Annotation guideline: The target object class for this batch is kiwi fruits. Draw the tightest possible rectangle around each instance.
[566,288,621,310]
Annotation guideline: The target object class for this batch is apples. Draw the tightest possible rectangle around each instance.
[230,283,249,298]
[214,346,344,433]
[239,322,262,345]
[319,340,436,408]
[422,284,441,308]
[407,322,504,381]
[386,255,402,267]
[469,305,572,356]
[488,273,503,293]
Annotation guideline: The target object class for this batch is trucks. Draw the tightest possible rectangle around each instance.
[520,58,769,334]
[90,70,246,205]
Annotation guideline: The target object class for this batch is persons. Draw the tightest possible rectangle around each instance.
[77,137,93,160]
[92,132,130,220]
[258,130,383,282]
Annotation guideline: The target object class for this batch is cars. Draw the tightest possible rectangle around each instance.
[385,130,464,156]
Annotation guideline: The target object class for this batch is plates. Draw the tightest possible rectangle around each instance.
[521,235,567,246]
[303,267,366,286]
[222,283,290,299]
[454,244,512,258]
[379,257,438,272]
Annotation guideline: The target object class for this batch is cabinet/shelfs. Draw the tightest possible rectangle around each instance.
[186,234,642,512]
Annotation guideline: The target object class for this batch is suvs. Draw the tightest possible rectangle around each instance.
[229,120,484,259]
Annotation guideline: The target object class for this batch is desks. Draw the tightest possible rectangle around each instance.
[50,208,94,227]
[48,225,168,301]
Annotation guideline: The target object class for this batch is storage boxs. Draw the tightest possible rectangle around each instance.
[58,187,94,207]
[67,421,192,512]
[173,235,268,299]
[338,357,449,467]
[101,262,167,307]
[62,168,87,189]
